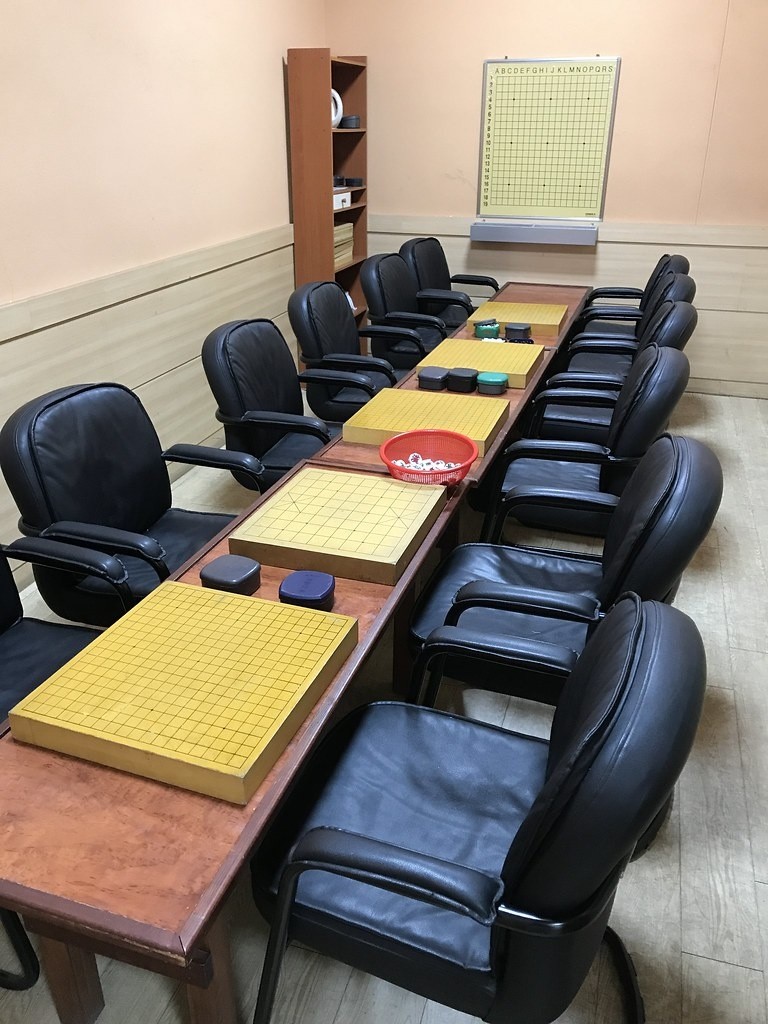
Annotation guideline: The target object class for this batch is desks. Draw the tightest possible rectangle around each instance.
[0,280,594,1023]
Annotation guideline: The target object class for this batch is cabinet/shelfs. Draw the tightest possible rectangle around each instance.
[286,47,369,389]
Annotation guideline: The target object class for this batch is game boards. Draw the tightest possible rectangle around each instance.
[227,466,449,587]
[467,301,569,337]
[7,578,359,806]
[416,338,545,389]
[343,387,511,458]
[475,56,622,222]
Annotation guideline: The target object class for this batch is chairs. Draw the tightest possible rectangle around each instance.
[250,253,725,1023]
[0,235,500,993]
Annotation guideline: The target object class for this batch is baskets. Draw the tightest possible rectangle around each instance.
[379,429,479,486]
